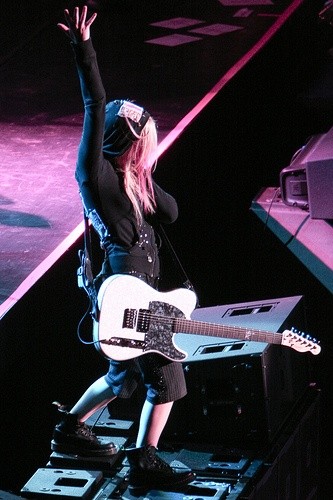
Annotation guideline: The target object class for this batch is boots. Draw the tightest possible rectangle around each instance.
[50,401,117,457]
[120,443,197,496]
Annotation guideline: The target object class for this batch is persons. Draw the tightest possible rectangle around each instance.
[50,5,196,496]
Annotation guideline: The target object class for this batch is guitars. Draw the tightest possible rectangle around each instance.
[93,273,322,362]
